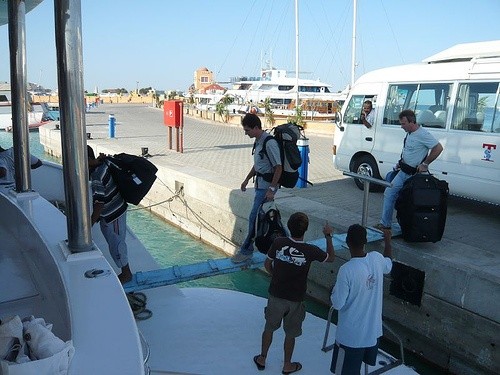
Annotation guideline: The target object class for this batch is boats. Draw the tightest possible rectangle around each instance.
[0,92,54,129]
[195,0,418,120]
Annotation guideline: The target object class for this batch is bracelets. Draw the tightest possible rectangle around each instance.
[268,186,276,192]
[422,162,428,168]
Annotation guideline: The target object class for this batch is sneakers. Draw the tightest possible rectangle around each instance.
[231,252,253,262]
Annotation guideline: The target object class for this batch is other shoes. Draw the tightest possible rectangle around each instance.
[118,272,132,285]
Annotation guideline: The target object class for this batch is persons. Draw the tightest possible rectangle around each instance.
[360,100,375,128]
[231,113,282,265]
[329,223,393,375]
[86,145,133,283]
[371,109,443,230]
[253,212,335,374]
[0,146,43,184]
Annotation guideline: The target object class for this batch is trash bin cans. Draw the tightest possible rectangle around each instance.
[296,137,310,189]
[109,113,115,138]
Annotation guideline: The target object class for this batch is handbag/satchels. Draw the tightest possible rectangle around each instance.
[386,170,399,183]
[0,314,75,375]
[394,169,449,243]
[101,153,158,206]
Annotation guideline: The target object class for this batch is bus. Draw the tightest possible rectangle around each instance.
[332,41,500,206]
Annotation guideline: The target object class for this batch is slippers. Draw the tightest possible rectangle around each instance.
[254,355,265,370]
[282,362,302,375]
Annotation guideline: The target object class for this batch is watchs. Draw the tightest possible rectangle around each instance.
[325,233,333,238]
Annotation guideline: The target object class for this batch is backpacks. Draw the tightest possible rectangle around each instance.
[244,197,289,255]
[252,121,304,188]
[399,161,417,175]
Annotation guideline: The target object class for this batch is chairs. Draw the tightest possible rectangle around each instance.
[383,112,401,125]
[480,108,500,133]
[415,109,484,130]
